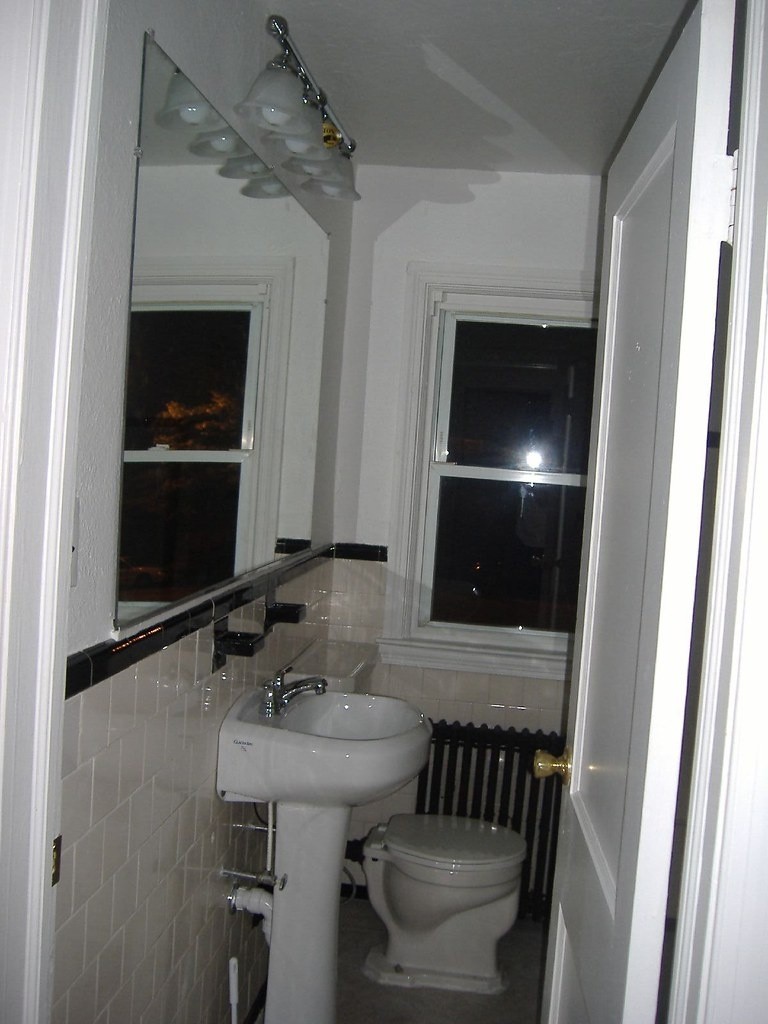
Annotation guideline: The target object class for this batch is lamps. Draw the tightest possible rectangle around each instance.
[261,85,332,163]
[299,143,362,203]
[232,48,312,138]
[281,132,345,184]
[155,69,293,200]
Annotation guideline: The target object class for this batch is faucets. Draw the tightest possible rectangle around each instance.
[274,678,327,716]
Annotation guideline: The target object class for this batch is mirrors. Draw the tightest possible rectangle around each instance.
[110,31,331,632]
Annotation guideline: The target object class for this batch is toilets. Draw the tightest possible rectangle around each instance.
[361,813,529,994]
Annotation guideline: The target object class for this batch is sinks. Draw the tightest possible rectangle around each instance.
[277,690,433,805]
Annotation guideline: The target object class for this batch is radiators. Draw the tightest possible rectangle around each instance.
[416,718,566,924]
